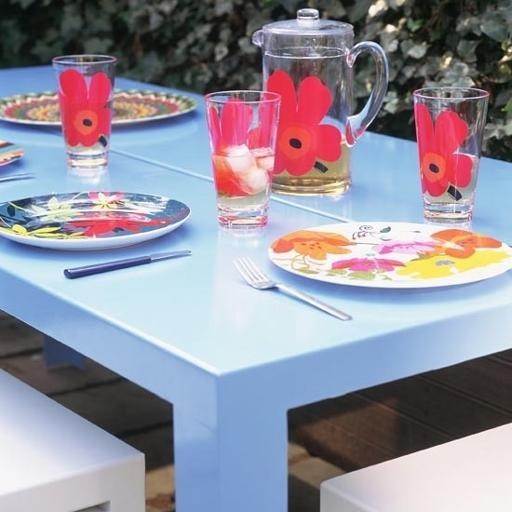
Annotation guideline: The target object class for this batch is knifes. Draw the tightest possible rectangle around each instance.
[0,171,36,182]
[63,248,193,279]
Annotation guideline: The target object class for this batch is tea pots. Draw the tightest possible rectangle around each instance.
[252,8,388,197]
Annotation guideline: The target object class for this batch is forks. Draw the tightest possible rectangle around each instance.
[231,255,353,322]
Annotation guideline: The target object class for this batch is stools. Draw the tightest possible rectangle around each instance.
[318,424,510,510]
[0,368,147,509]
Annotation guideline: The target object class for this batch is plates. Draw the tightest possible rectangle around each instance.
[268,220,512,291]
[0,86,199,128]
[0,137,26,168]
[0,190,192,251]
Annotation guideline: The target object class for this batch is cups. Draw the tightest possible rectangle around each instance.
[412,85,490,222]
[52,54,117,168]
[205,89,284,228]
